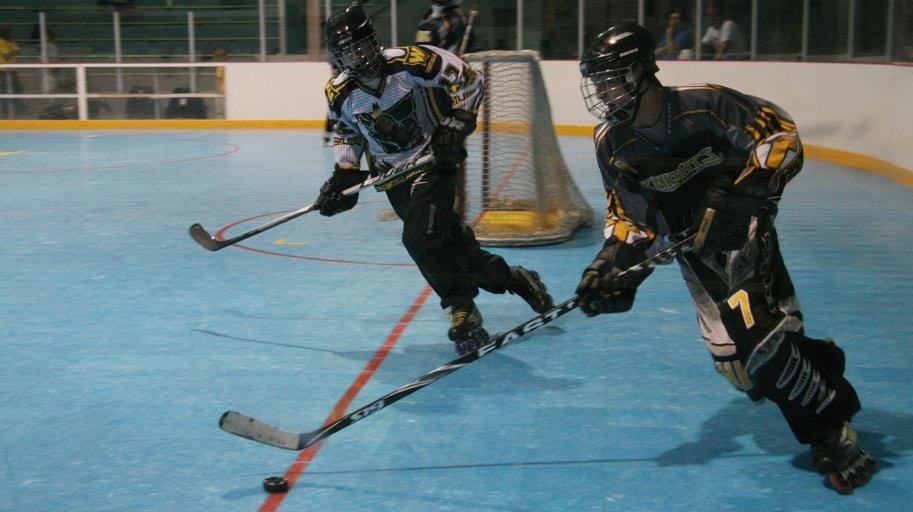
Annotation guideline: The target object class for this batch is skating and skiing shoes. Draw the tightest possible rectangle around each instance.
[505,263,554,315]
[807,422,878,495]
[445,299,490,356]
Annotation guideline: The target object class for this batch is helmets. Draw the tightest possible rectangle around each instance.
[576,20,659,130]
[321,3,384,78]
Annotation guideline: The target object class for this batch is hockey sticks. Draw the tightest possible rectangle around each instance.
[217,232,698,449]
[189,150,434,253]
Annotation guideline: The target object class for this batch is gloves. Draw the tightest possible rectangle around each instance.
[428,106,478,168]
[313,160,370,218]
[573,234,653,319]
[668,183,764,255]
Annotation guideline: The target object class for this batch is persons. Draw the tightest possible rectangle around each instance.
[317,5,548,342]
[575,21,865,473]
[0,0,912,124]
